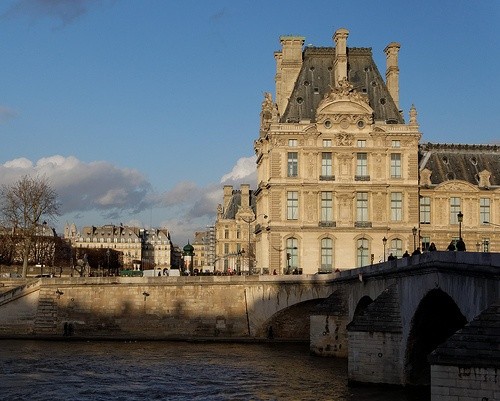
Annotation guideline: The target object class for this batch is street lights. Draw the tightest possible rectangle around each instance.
[456,210,463,240]
[235,213,268,276]
[383,236,387,262]
[360,245,364,268]
[412,227,418,251]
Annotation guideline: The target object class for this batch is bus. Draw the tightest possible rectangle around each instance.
[120,270,144,278]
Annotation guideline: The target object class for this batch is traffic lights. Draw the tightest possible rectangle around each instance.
[287,253,291,260]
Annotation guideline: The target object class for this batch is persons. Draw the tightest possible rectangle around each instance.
[262,92,273,111]
[59,268,63,278]
[409,104,417,122]
[217,203,222,218]
[446,238,466,251]
[188,269,237,276]
[428,241,437,252]
[330,76,369,103]
[273,267,303,275]
[388,247,421,262]
